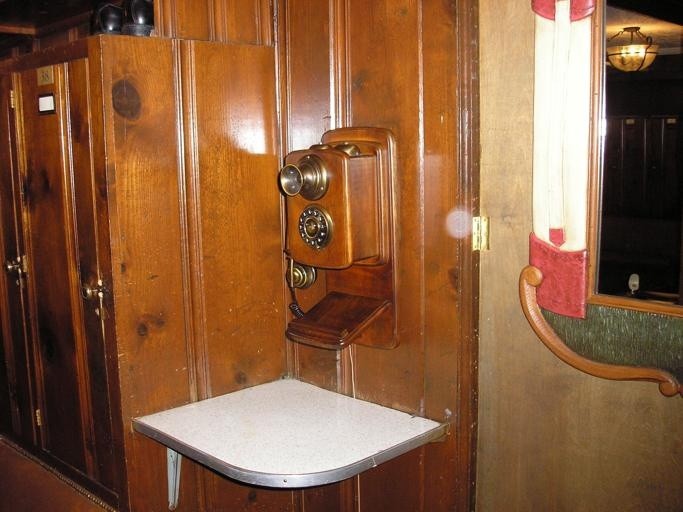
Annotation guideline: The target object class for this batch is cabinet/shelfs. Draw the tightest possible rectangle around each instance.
[0,61,39,452]
[10,35,296,512]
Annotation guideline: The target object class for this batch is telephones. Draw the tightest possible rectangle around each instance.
[278,126,398,318]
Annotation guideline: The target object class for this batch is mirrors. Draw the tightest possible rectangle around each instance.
[586,0,683,318]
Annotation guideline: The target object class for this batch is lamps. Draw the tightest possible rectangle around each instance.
[607,27,658,72]
